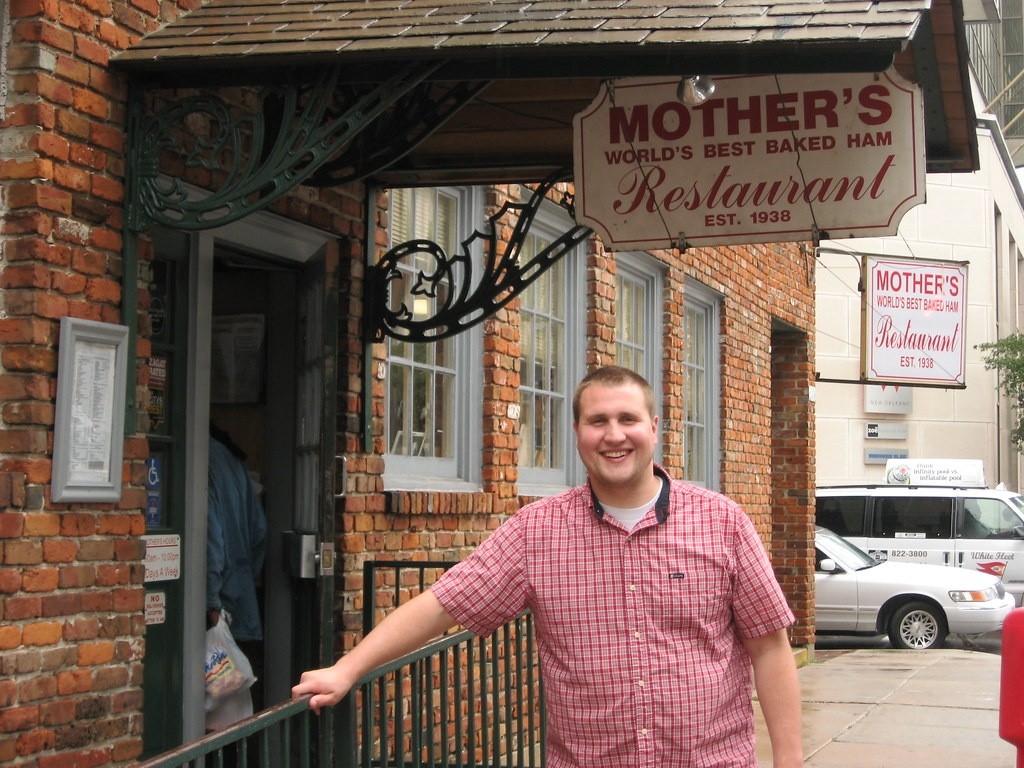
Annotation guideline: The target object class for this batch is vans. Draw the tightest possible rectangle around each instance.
[814,459,1024,608]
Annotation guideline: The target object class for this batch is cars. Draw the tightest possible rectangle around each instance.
[813,525,1016,650]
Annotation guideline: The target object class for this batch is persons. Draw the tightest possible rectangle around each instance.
[205,436,270,642]
[291,366,802,768]
